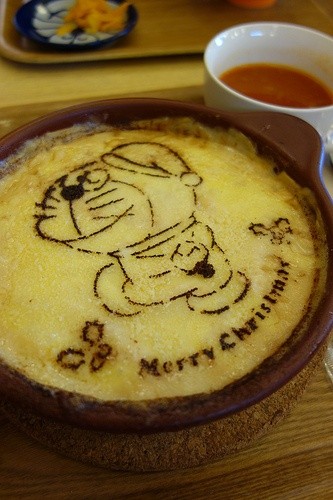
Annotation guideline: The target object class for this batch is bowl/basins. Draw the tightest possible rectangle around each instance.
[0,98,333,432]
[203,21,333,137]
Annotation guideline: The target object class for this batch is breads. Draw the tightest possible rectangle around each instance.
[1,115,329,401]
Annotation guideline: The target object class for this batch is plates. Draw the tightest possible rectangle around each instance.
[14,0,137,51]
